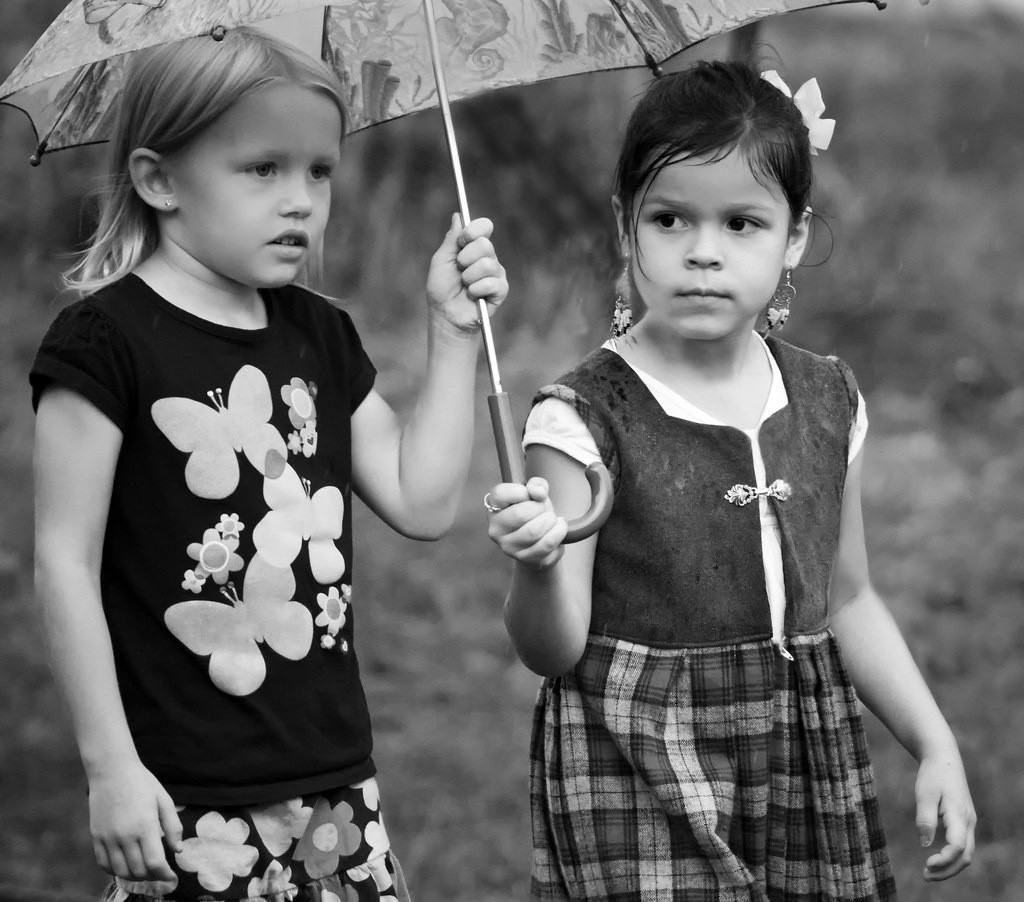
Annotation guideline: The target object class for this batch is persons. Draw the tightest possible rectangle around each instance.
[486,60,978,901]
[27,25,510,902]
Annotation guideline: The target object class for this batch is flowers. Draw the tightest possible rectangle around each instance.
[759,66,838,157]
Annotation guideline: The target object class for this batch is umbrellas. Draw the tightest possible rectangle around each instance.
[1,1,894,541]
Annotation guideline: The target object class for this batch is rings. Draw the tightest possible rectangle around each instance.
[483,493,501,513]
